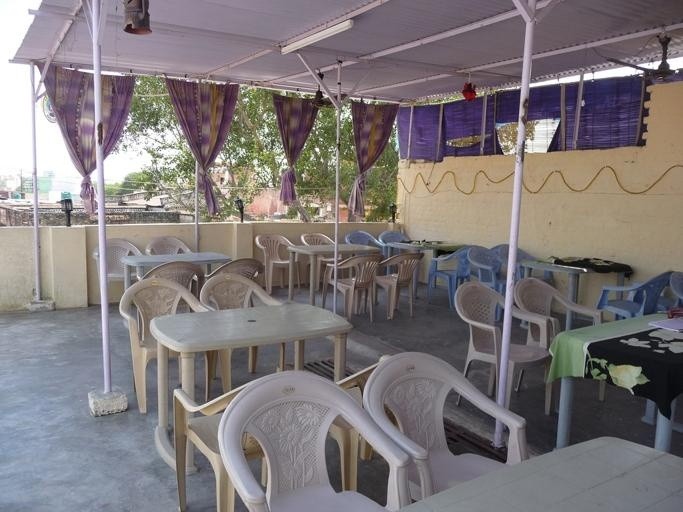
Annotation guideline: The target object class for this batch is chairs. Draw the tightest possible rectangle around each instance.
[514,277,607,403]
[345,230,383,249]
[294,232,337,295]
[118,276,216,416]
[137,259,204,313]
[597,270,675,319]
[362,353,531,500]
[467,245,506,322]
[378,230,412,257]
[321,254,384,325]
[146,236,198,275]
[199,272,285,394]
[217,370,411,512]
[387,240,423,299]
[172,375,259,479]
[255,235,295,295]
[453,282,552,433]
[204,258,265,306]
[93,237,143,312]
[427,245,470,307]
[373,252,424,321]
[329,362,393,480]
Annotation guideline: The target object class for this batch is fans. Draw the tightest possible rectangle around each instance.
[303,72,348,110]
[606,37,682,81]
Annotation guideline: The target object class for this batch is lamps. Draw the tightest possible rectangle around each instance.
[234,196,246,221]
[388,202,396,222]
[122,1,153,35]
[280,19,354,56]
[60,192,74,225]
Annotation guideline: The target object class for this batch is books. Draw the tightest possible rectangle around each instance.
[649,314,682,333]
